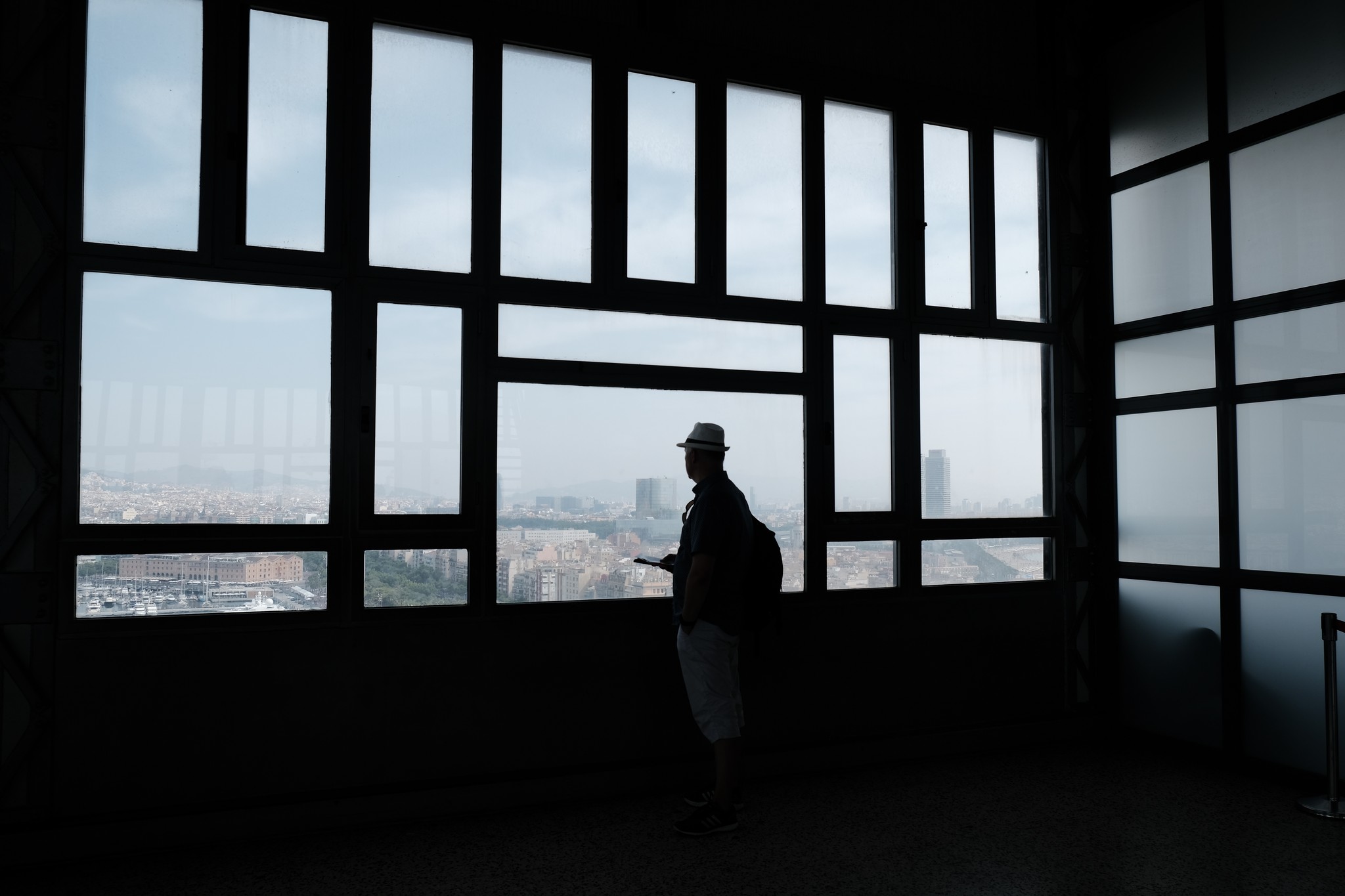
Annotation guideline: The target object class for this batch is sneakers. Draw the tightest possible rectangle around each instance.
[684,784,744,811]
[672,803,738,835]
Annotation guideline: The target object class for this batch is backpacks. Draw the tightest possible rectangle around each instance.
[697,481,783,629]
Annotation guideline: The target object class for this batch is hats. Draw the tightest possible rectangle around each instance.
[677,422,730,452]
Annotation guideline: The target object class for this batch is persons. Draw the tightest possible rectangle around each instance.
[654,423,753,835]
